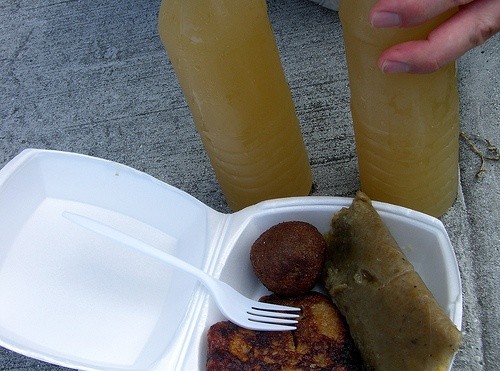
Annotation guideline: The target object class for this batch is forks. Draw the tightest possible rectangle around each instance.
[62,211,301,331]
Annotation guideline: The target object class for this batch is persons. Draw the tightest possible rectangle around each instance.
[370,1,499,73]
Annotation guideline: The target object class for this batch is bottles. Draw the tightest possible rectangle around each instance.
[158,0,311,210]
[339,1,459,217]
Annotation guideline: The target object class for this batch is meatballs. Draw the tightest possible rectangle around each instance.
[250,221,327,298]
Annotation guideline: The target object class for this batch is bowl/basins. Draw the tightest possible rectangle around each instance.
[176,195,462,371]
